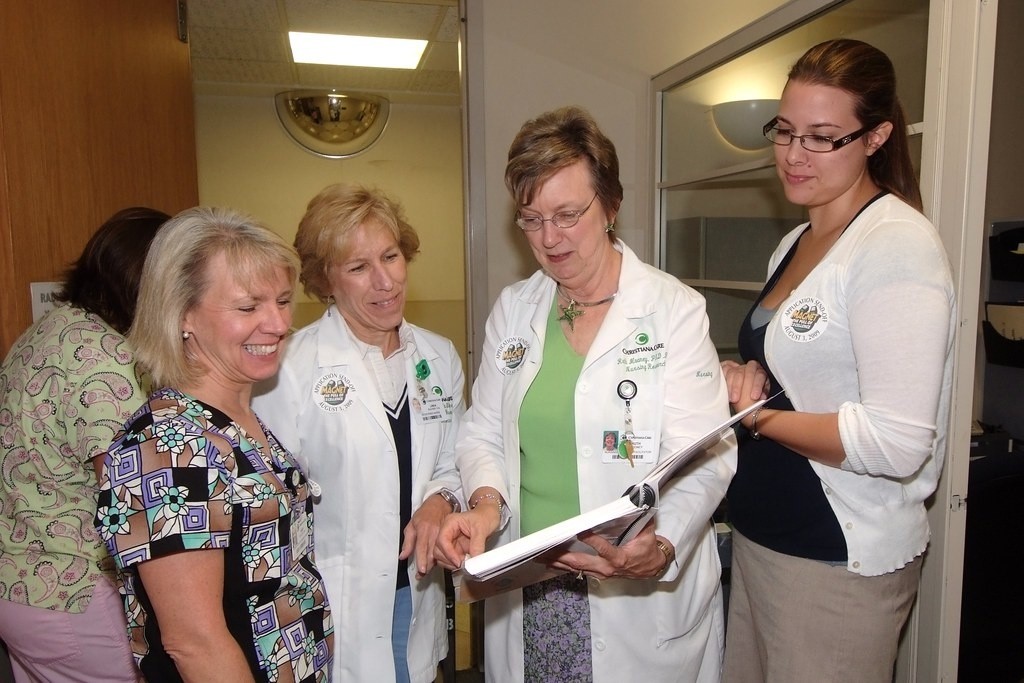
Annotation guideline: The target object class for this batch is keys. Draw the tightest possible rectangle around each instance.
[626,441,634,467]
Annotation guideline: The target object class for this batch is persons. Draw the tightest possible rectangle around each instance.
[99,205,333,683]
[413,398,424,415]
[251,184,467,683]
[718,40,957,682]
[433,106,739,683]
[0,207,171,683]
[603,432,618,453]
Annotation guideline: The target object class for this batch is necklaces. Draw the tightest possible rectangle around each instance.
[557,285,616,331]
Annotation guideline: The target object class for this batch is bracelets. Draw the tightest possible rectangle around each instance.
[471,494,503,517]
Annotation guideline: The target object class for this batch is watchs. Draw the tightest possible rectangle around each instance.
[751,407,767,440]
[439,490,460,514]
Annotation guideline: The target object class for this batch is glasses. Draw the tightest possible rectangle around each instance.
[514,193,599,232]
[763,115,876,152]
